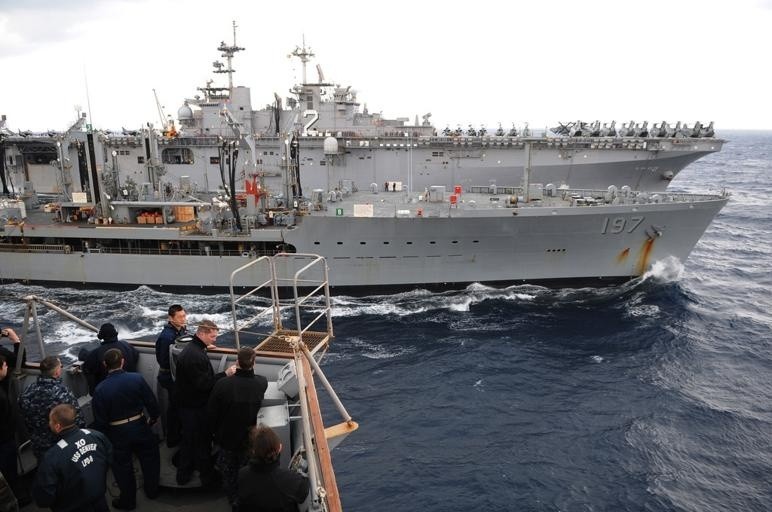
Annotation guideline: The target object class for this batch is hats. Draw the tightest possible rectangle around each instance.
[96,323,118,338]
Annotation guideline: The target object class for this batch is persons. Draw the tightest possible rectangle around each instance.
[1,304,310,511]
[392,182,396,192]
[384,181,389,192]
